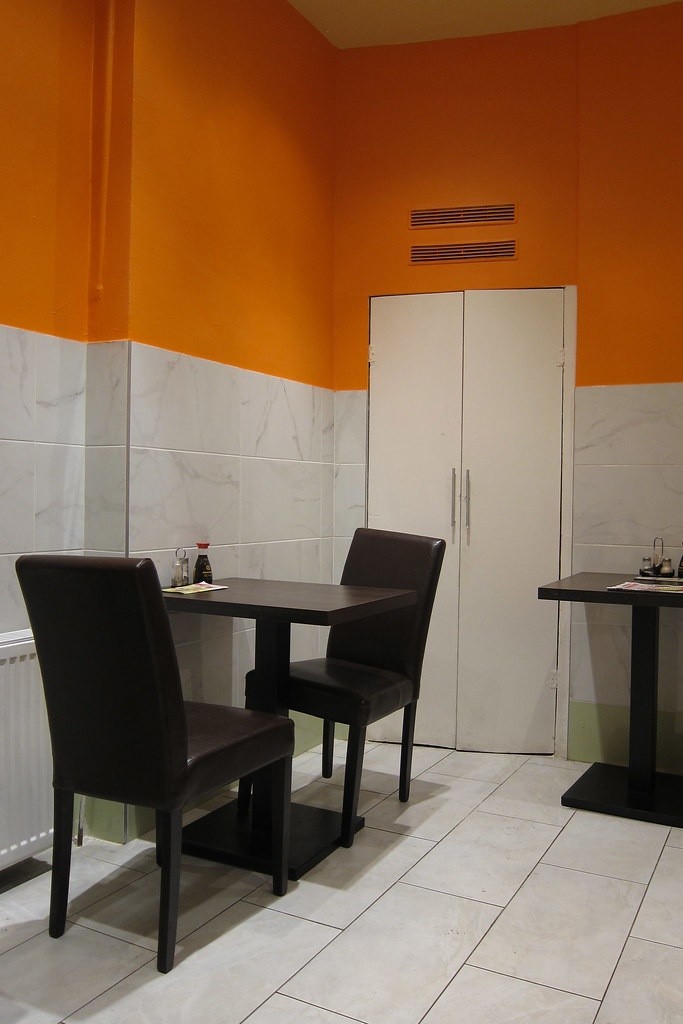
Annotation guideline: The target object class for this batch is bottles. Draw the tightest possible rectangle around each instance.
[678,541,683,578]
[640,556,652,571]
[192,543,213,584]
[660,558,672,574]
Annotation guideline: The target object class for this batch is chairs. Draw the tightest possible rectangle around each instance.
[14,554,296,974]
[245,527,447,848]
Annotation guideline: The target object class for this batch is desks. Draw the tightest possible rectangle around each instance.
[158,577,418,882]
[538,572,683,829]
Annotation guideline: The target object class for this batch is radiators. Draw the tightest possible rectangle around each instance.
[0,628,52,872]
